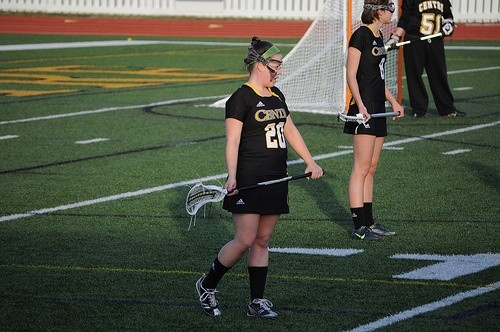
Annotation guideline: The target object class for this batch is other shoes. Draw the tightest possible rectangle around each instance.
[446,110,466,119]
[413,111,426,118]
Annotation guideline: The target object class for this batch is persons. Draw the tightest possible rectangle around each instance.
[196,36,323,318]
[345,0,404,241]
[385,0,467,117]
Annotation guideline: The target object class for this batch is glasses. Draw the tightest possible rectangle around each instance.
[364,2,395,13]
[267,59,283,68]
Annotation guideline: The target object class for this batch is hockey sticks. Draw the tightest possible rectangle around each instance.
[185,167,325,215]
[337,110,400,124]
[384,32,442,51]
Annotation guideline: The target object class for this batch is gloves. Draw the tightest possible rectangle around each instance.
[385,32,402,51]
[440,19,455,40]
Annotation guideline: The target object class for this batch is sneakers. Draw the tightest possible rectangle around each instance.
[247,298,279,318]
[197,273,222,316]
[353,226,382,240]
[372,222,396,235]
